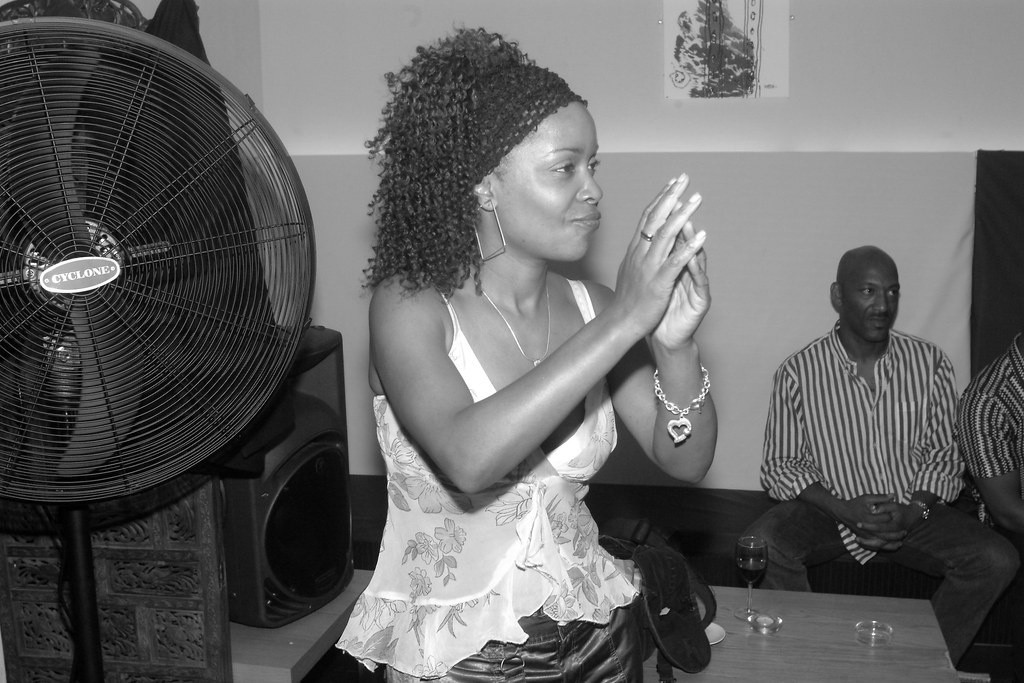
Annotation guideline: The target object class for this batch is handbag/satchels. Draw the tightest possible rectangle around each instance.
[598,516,717,683]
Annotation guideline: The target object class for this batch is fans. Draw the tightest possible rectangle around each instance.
[0,15,316,683]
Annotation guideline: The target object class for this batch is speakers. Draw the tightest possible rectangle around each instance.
[218,326,356,628]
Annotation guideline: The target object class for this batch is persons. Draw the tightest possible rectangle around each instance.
[956,325,1024,532]
[334,24,717,683]
[740,246,1018,666]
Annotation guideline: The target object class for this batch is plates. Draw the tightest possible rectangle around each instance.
[706,623,726,645]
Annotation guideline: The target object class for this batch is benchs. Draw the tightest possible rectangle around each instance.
[592,478,1023,646]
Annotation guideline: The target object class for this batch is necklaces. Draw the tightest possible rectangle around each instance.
[469,273,551,368]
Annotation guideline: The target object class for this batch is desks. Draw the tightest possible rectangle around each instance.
[233,565,377,683]
[634,584,967,683]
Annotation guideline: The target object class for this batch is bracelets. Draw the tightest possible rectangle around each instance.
[653,365,710,444]
[912,499,930,520]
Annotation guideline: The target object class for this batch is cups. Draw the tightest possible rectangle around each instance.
[854,590,893,646]
[749,586,782,633]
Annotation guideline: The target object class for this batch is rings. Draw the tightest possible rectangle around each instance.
[640,231,653,242]
[888,512,893,520]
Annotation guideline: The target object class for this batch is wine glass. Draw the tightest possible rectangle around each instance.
[734,536,768,621]
